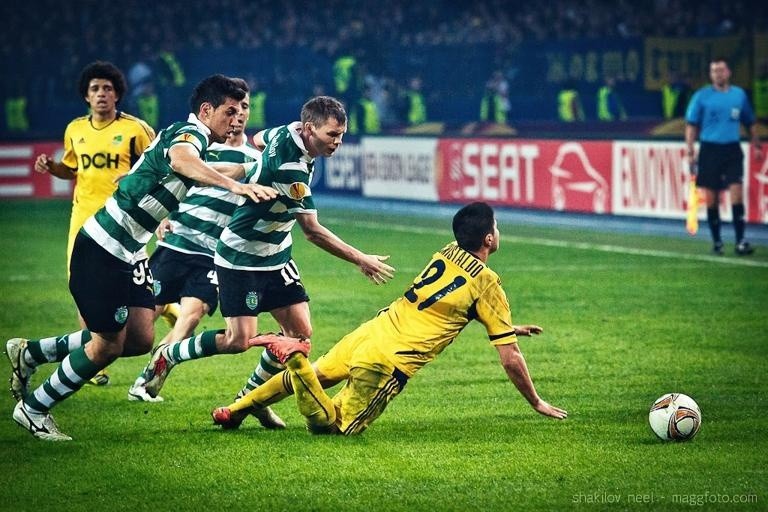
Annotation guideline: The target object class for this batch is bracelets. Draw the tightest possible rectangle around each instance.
[686,151,693,155]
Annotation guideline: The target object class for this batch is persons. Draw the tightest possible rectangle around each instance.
[4,75,280,442]
[684,58,756,256]
[210,200,568,436]
[125,94,396,427]
[1,1,767,149]
[105,77,264,402]
[33,61,180,387]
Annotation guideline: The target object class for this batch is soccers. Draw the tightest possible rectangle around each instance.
[649,393,701,440]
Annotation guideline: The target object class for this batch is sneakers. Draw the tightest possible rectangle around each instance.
[212,407,242,430]
[7,339,34,400]
[14,400,73,443]
[253,408,287,430]
[84,371,108,385]
[249,333,310,363]
[714,243,724,253]
[127,387,162,403]
[736,240,753,254]
[147,345,172,399]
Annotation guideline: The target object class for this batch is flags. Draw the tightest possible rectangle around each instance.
[686,172,700,236]
